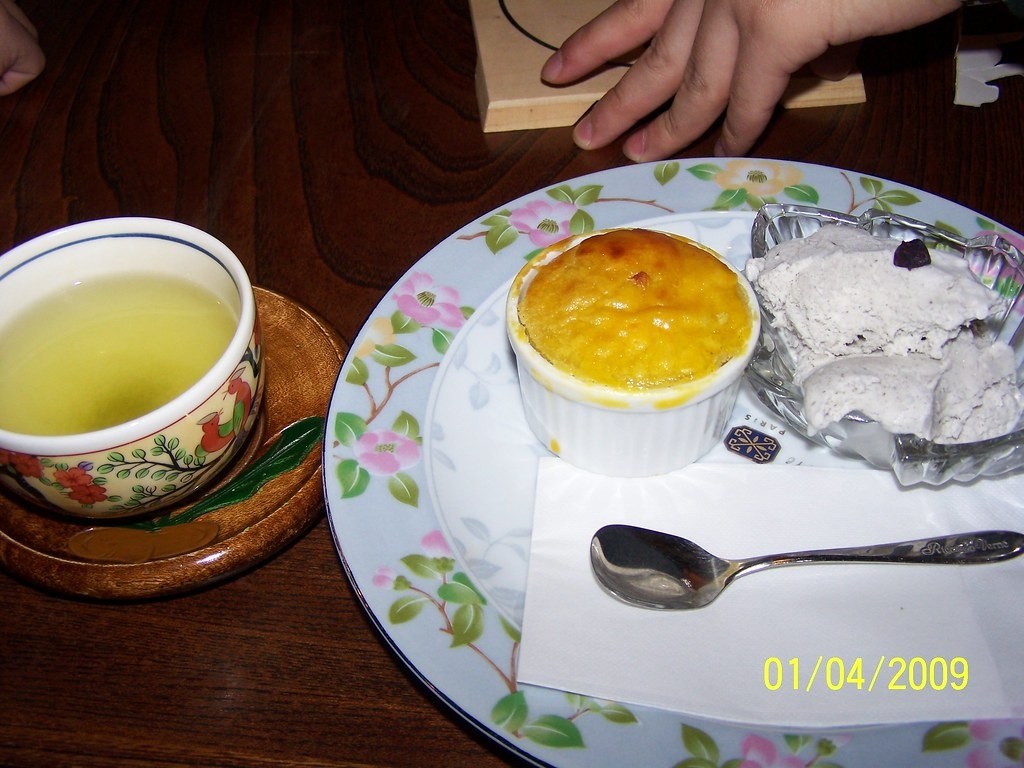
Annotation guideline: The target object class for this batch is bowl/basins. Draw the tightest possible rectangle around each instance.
[0,218,262,520]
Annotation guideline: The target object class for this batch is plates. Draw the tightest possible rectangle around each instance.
[744,205,1024,484]
[322,157,1024,768]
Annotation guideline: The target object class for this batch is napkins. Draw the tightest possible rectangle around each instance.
[517,449,1024,718]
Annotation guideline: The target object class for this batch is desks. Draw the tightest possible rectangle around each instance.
[0,0,1024,768]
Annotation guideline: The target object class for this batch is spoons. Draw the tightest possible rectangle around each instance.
[590,525,1024,611]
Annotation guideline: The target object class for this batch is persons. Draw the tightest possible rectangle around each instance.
[539,0,994,161]
[0,0,46,97]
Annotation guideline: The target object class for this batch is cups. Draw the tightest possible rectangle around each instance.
[507,230,761,474]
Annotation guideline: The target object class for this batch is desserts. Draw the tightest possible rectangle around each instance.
[504,226,761,477]
[741,218,1021,449]
